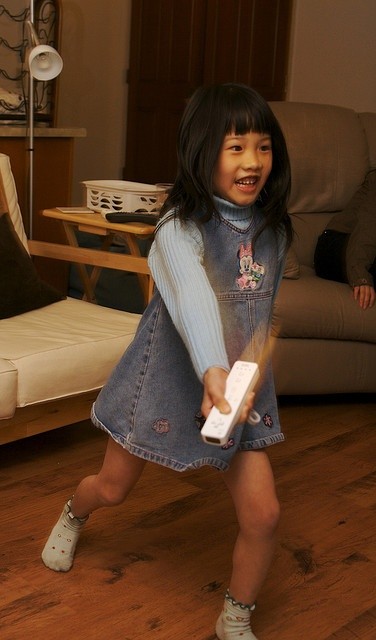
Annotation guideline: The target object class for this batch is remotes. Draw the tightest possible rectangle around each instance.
[106,212,157,225]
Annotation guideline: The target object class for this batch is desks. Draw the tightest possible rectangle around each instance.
[40,203,156,313]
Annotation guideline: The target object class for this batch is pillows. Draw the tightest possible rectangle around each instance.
[0,211,69,323]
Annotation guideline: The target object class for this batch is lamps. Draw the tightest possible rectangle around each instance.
[24,42,66,239]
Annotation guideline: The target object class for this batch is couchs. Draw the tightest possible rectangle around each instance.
[0,152,145,448]
[254,99,375,401]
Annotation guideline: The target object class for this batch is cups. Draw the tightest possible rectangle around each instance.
[155,183,174,216]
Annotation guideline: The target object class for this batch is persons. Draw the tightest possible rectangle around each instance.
[313,169,376,310]
[41,83,299,640]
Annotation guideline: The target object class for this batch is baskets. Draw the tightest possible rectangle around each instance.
[81,179,166,215]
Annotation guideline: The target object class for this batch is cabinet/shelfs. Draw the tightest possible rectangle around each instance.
[1,123,88,263]
